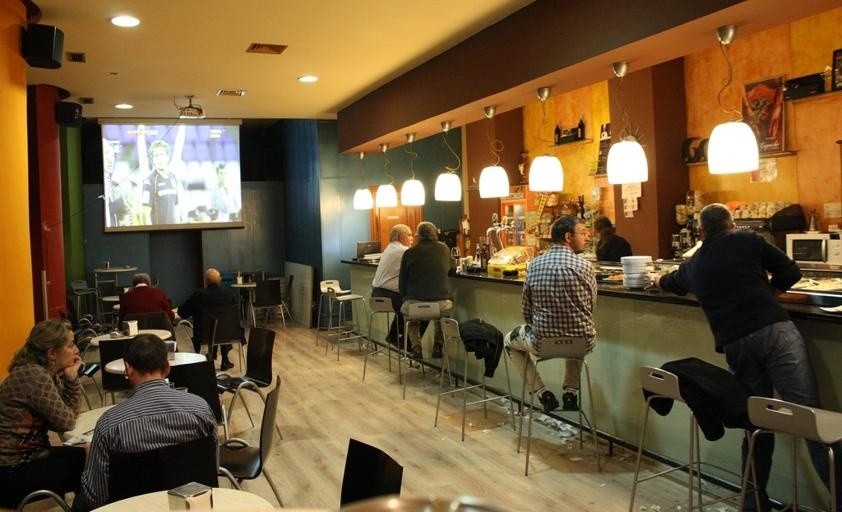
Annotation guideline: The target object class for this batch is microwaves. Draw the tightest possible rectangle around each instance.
[785,232,842,266]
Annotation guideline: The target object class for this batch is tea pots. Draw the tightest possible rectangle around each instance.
[105,327,125,338]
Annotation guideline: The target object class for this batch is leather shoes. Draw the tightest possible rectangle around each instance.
[222,362,234,371]
[206,354,217,360]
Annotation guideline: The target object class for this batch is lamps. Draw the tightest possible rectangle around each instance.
[478,104,511,199]
[526,87,565,193]
[352,120,463,211]
[605,61,650,186]
[706,24,759,176]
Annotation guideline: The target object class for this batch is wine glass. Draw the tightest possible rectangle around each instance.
[451,247,461,266]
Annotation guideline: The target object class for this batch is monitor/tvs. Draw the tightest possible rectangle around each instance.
[357,241,381,258]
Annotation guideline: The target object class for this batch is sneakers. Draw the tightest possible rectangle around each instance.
[563,392,578,411]
[386,334,411,351]
[432,343,442,358]
[409,346,421,359]
[538,391,559,412]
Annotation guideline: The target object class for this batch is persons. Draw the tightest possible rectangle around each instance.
[72,333,222,511]
[118,273,175,331]
[643,202,841,511]
[398,221,457,359]
[593,216,632,262]
[178,268,247,371]
[103,138,137,227]
[141,140,182,224]
[504,214,597,412]
[1,319,92,512]
[372,223,430,352]
[213,162,240,212]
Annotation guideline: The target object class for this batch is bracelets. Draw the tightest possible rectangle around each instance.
[650,276,657,288]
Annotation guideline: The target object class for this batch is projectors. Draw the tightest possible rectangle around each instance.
[177,107,207,119]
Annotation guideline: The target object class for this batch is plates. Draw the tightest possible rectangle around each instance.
[599,255,655,290]
[680,137,709,163]
[364,254,381,260]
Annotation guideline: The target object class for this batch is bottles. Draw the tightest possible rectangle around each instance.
[235,270,253,285]
[518,152,530,182]
[103,261,110,270]
[823,62,832,92]
[600,123,611,139]
[734,200,790,218]
[576,195,585,222]
[554,123,560,144]
[461,212,517,275]
[577,118,585,138]
[807,207,819,231]
[672,189,704,261]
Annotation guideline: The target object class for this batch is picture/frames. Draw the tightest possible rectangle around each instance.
[739,74,788,154]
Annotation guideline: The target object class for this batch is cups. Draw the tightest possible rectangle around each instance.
[164,378,188,393]
[352,256,358,260]
